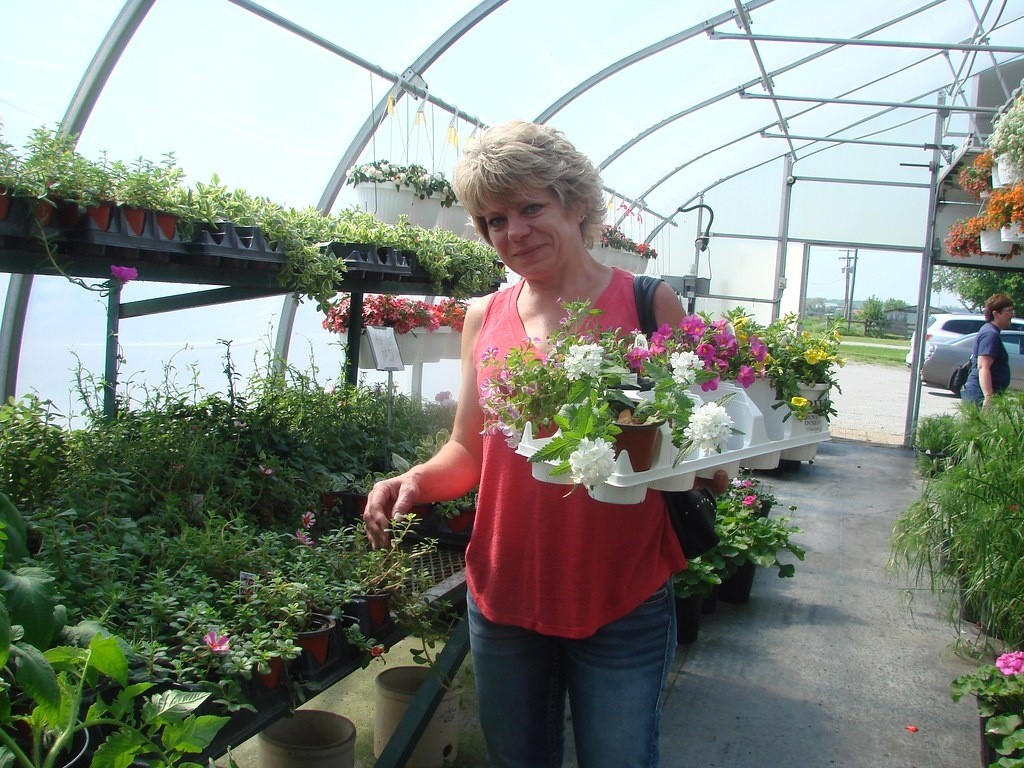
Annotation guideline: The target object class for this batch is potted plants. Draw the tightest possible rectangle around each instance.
[0,142,845,768]
[913,98,1024,767]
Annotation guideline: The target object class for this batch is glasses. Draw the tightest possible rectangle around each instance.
[1002,309,1013,313]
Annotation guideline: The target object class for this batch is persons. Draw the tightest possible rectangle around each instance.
[360,120,725,768]
[954,295,1015,410]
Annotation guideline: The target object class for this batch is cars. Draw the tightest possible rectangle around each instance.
[920,328,1024,398]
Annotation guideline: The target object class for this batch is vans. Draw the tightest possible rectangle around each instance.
[904,312,1023,368]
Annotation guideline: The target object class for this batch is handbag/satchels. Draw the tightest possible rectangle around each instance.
[664,488,721,561]
